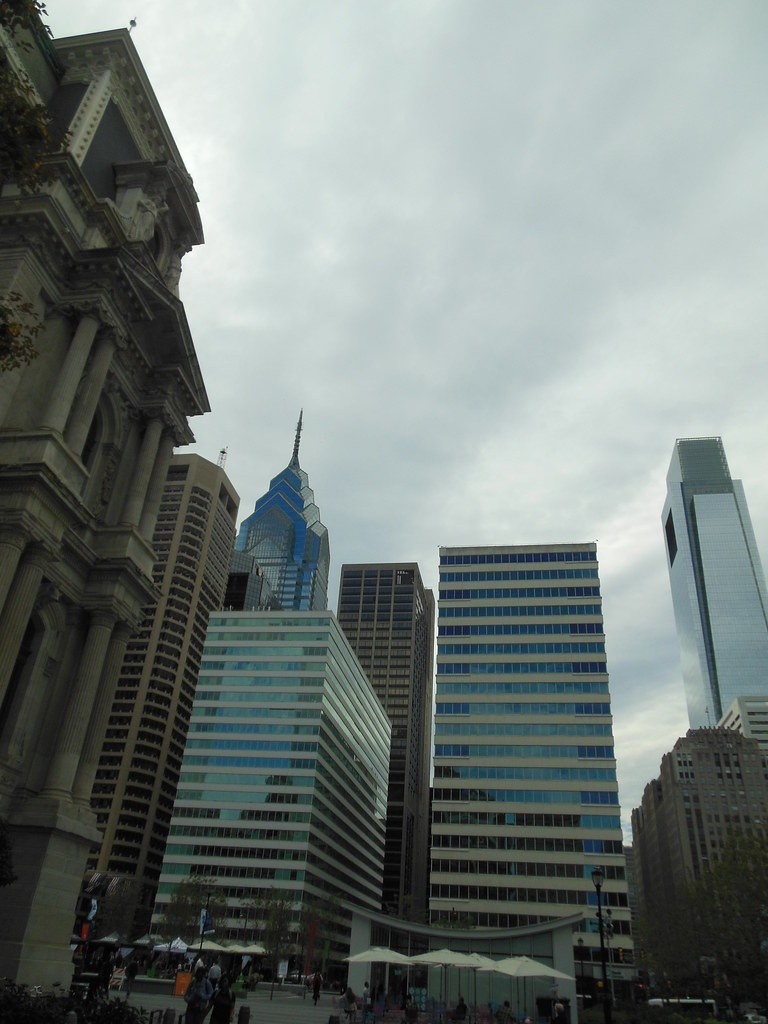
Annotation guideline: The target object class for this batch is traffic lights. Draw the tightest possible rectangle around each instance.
[639,948,647,962]
[617,946,625,961]
[638,971,647,989]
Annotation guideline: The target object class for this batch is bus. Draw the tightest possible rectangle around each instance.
[647,996,718,1024]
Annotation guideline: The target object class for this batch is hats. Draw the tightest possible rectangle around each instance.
[560,997,568,1005]
[556,1003,564,1014]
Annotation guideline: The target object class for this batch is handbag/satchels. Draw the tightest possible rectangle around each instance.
[366,997,371,1006]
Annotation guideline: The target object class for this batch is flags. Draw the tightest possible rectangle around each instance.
[103,877,127,896]
[84,873,107,896]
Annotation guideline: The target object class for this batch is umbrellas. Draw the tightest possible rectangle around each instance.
[342,946,576,1023]
[91,931,273,955]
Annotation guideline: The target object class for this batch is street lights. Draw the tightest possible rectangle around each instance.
[589,909,616,1004]
[198,886,216,958]
[576,937,586,1009]
[591,865,615,1024]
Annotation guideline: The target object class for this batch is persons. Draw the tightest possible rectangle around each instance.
[305,972,327,1006]
[80,941,253,1024]
